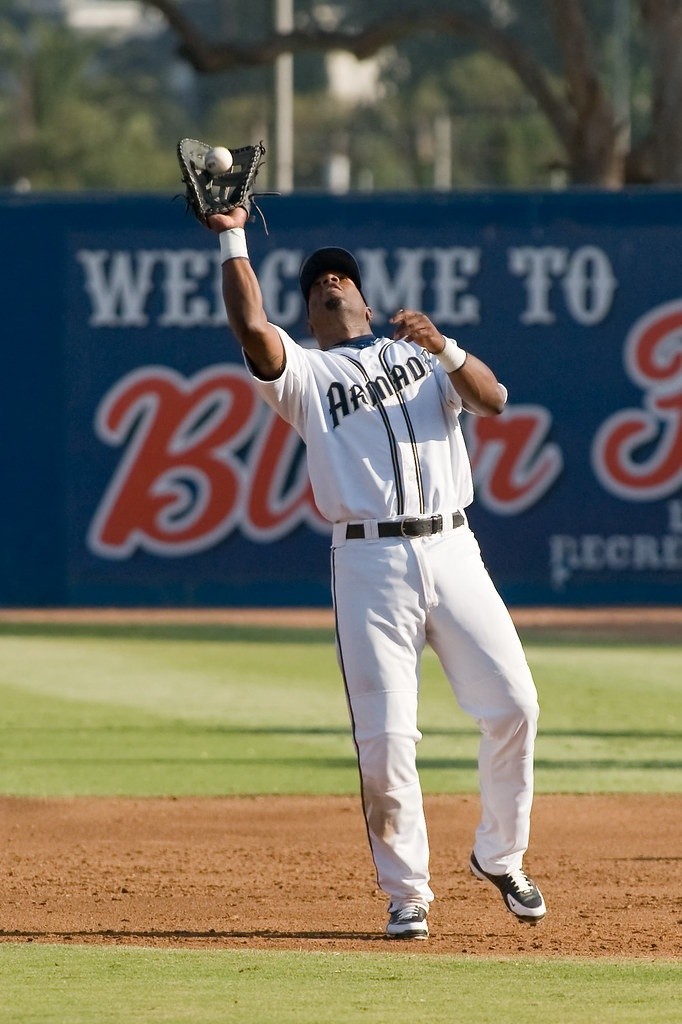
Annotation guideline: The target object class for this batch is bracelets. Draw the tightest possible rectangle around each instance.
[434,335,467,375]
[218,227,249,265]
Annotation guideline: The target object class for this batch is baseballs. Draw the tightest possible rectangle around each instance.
[205,145,233,174]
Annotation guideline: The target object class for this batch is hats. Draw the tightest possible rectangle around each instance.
[297,246,361,302]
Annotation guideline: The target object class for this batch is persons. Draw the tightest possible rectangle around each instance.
[181,140,549,939]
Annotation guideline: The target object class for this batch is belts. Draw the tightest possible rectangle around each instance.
[346,511,464,539]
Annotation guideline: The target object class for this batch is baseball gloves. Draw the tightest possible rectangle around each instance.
[170,137,283,236]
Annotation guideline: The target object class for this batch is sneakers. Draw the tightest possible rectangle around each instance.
[383,903,429,942]
[469,850,547,924]
